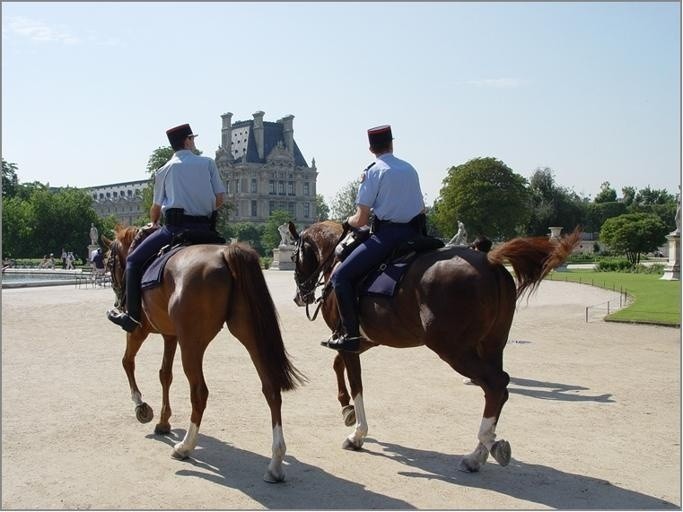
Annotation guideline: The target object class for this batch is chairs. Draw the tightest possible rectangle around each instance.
[75,267,111,289]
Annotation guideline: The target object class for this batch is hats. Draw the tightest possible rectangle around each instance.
[166,124,199,143]
[368,126,393,152]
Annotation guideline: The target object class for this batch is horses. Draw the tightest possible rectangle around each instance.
[288,219,581,474]
[100,222,312,484]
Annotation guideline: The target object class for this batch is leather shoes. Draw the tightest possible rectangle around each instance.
[106,309,137,332]
[321,334,360,352]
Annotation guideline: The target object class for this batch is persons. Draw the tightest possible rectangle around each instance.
[88,222,100,247]
[105,123,225,334]
[674,184,682,233]
[320,124,429,353]
[1,248,105,286]
[461,235,499,386]
[278,222,292,244]
